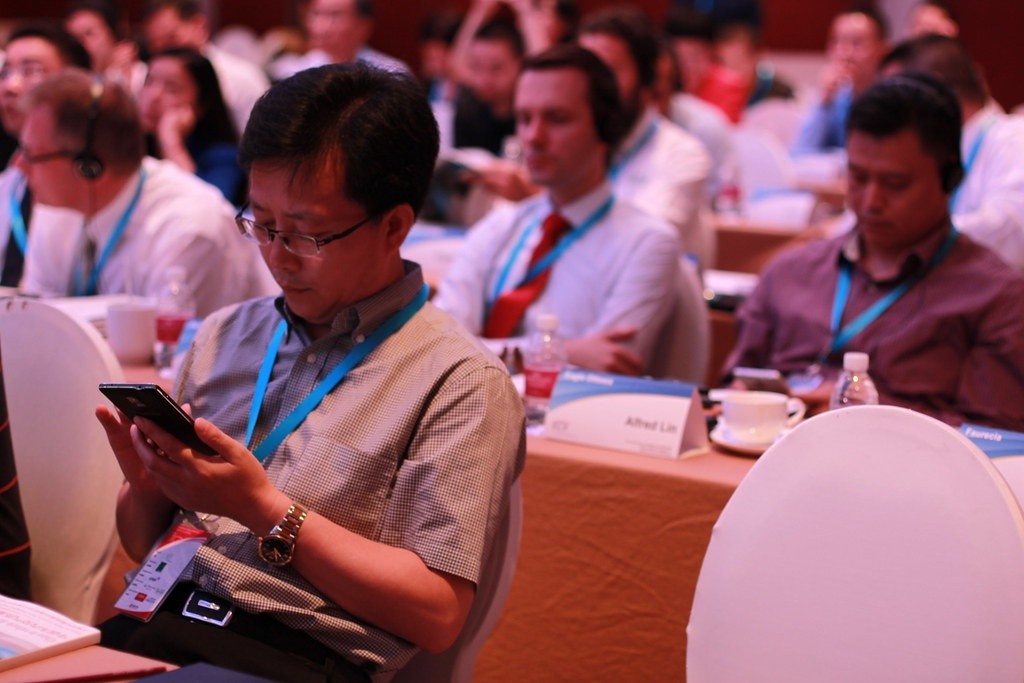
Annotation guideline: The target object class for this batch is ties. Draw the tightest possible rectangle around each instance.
[485,214,574,338]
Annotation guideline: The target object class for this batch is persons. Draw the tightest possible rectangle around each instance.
[722,74,1024,434]
[787,6,890,157]
[431,43,710,384]
[876,32,1024,264]
[96,62,527,683]
[60,0,270,136]
[465,9,718,267]
[578,15,717,268]
[15,66,282,320]
[623,0,794,194]
[0,20,92,287]
[270,0,409,82]
[139,45,249,211]
[408,0,564,157]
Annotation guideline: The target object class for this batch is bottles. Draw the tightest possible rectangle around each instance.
[151,265,197,381]
[523,314,568,426]
[829,352,878,412]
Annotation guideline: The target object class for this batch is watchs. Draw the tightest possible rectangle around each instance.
[258,502,309,565]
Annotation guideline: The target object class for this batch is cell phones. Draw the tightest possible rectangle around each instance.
[100,383,219,457]
[734,366,792,395]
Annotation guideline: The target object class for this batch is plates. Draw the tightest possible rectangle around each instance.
[711,428,778,454]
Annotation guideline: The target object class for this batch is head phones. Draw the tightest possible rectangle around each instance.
[882,79,964,192]
[584,49,623,145]
[72,82,104,180]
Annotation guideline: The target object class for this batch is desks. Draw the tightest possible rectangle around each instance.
[99,166,847,683]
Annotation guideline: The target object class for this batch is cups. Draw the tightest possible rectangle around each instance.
[720,391,806,444]
[106,302,155,363]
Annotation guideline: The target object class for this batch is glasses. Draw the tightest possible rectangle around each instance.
[234,188,375,259]
[17,139,81,170]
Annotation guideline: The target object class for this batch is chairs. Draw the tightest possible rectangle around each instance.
[687,406,1024,683]
[0,295,128,630]
[388,479,523,683]
[648,253,714,389]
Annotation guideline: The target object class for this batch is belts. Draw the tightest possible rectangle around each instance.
[160,582,373,683]
